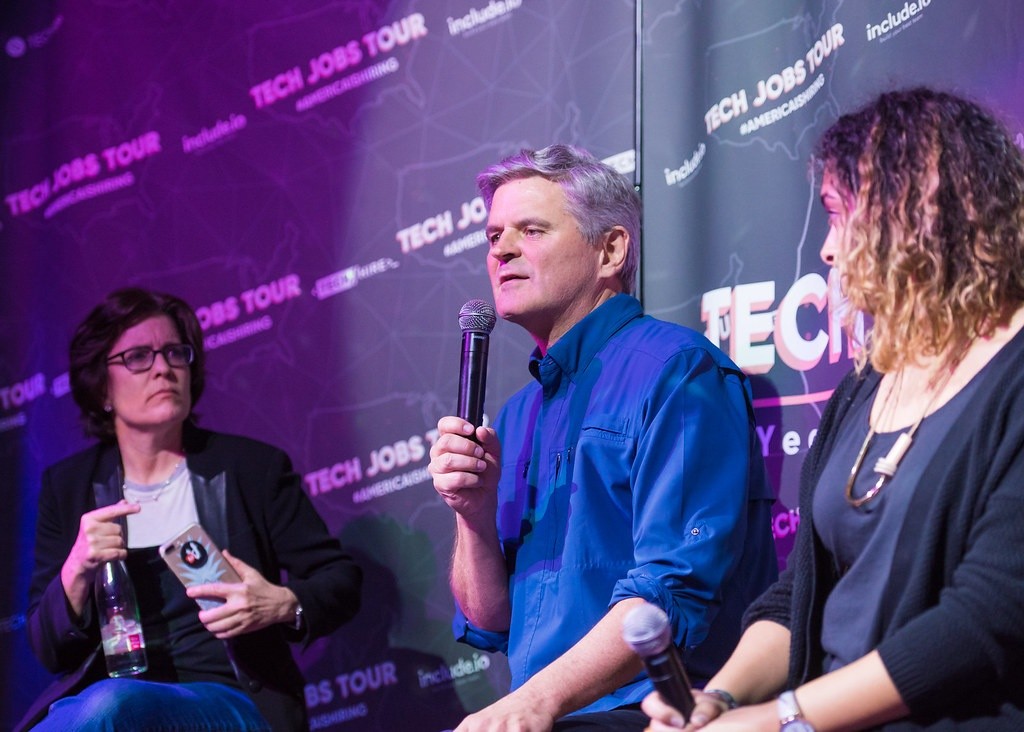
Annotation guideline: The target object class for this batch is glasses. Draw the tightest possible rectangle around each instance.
[107,344,195,371]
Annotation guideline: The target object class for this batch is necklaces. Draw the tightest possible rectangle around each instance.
[845,336,975,508]
[123,447,185,504]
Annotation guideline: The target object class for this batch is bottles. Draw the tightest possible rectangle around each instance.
[94,560,148,678]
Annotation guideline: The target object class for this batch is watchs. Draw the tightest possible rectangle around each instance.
[778,690,816,732]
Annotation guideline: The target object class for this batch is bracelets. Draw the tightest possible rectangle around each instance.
[289,605,305,631]
[704,689,737,710]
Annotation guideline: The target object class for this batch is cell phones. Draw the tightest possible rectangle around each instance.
[158,523,244,613]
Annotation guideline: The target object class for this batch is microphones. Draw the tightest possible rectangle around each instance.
[456,298,497,448]
[620,601,697,724]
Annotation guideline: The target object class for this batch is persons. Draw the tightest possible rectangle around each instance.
[641,86,1024,732]
[428,143,779,732]
[10,286,365,732]
